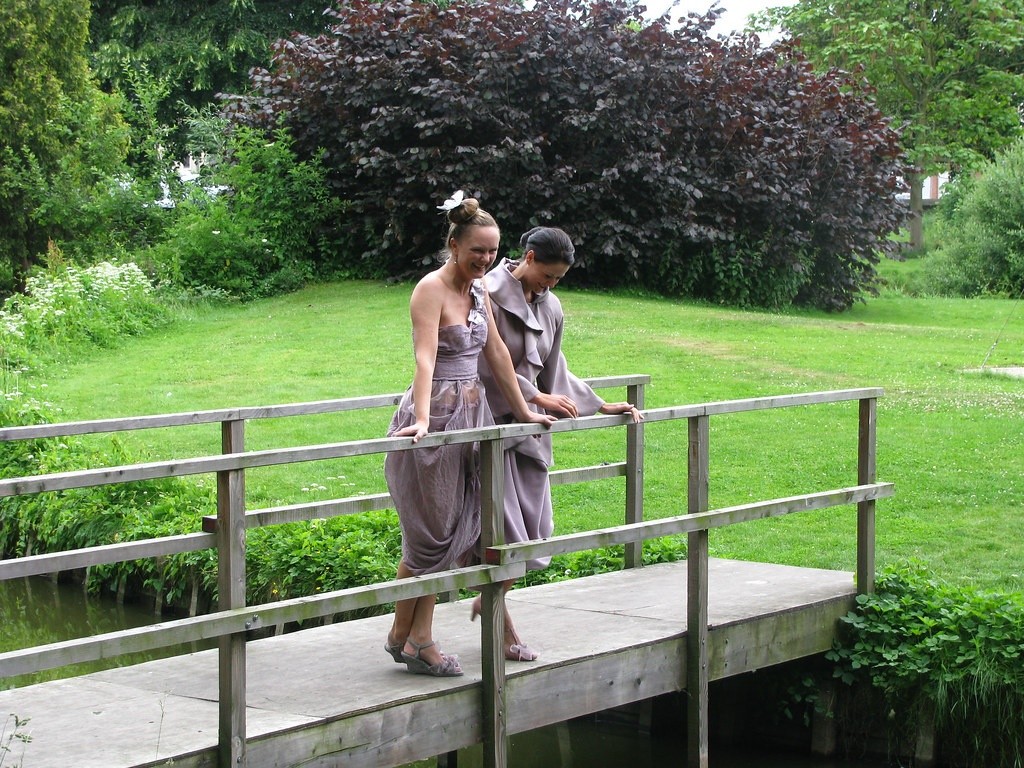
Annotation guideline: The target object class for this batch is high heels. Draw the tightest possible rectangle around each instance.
[400,637,464,677]
[384,633,444,663]
[504,626,540,661]
[470,593,481,622]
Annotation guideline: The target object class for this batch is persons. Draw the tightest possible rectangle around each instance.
[470,227,645,660]
[383,191,560,676]
